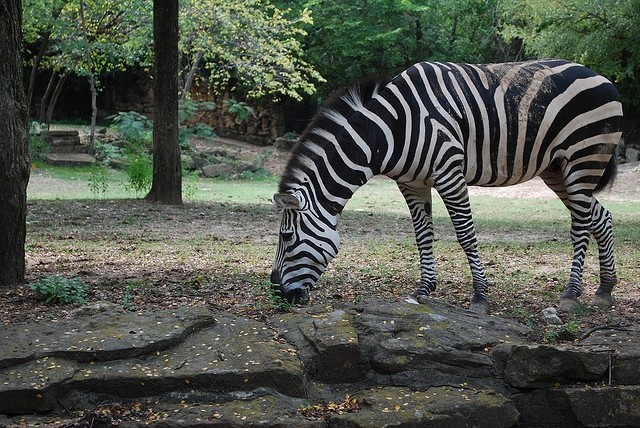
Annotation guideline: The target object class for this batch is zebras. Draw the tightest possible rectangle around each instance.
[269,57,625,308]
